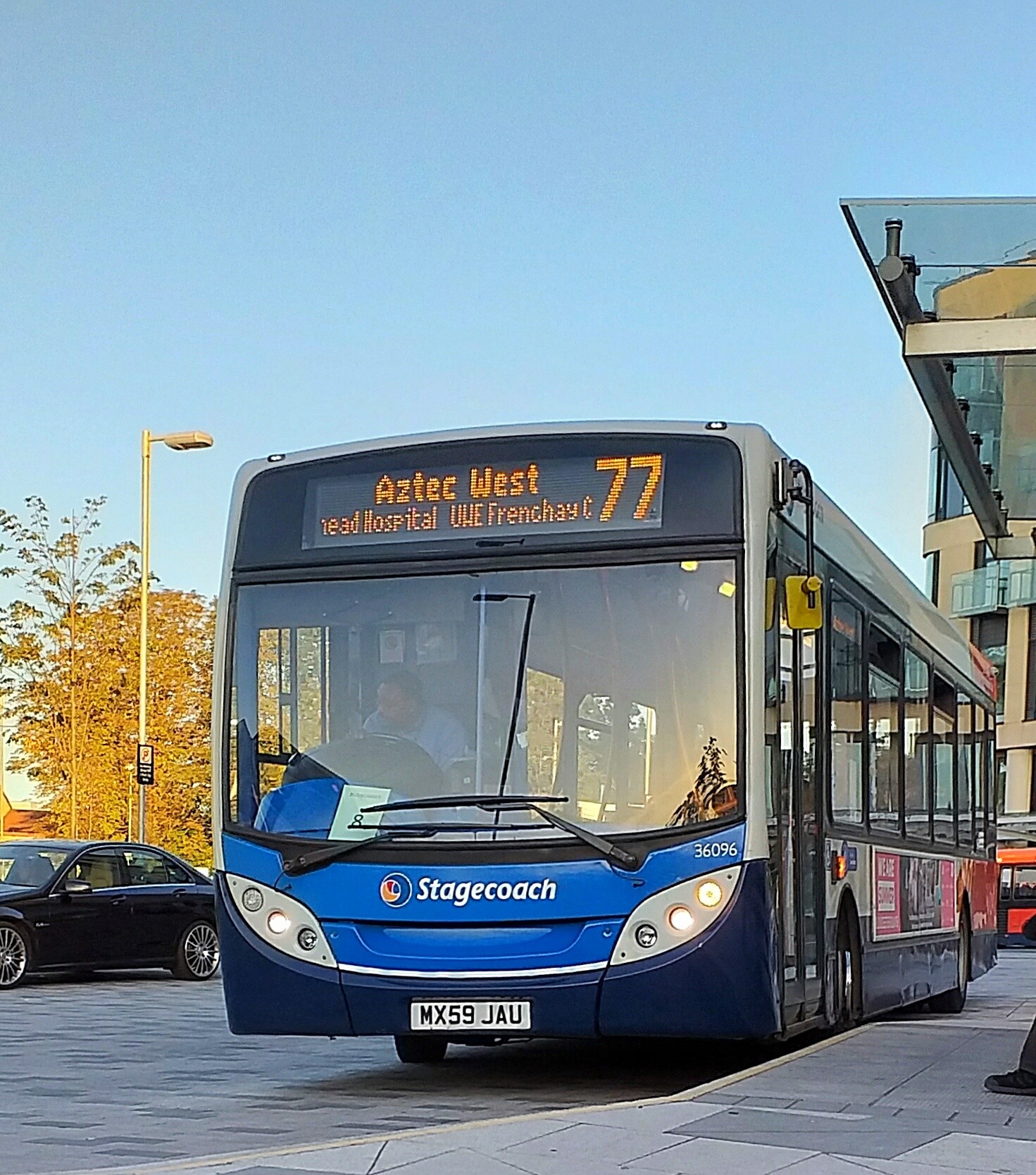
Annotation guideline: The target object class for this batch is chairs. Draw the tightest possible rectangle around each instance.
[43,855,170,890]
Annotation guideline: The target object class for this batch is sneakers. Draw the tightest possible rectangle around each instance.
[984,1068,1036,1094]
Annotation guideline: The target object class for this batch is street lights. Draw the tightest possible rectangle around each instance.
[137,429,214,844]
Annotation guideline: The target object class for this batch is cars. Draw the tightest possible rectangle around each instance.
[0,841,220,990]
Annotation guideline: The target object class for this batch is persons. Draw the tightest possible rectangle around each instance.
[15,857,40,884]
[346,672,465,788]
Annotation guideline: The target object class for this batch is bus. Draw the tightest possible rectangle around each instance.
[212,420,999,1065]
[995,847,1036,948]
